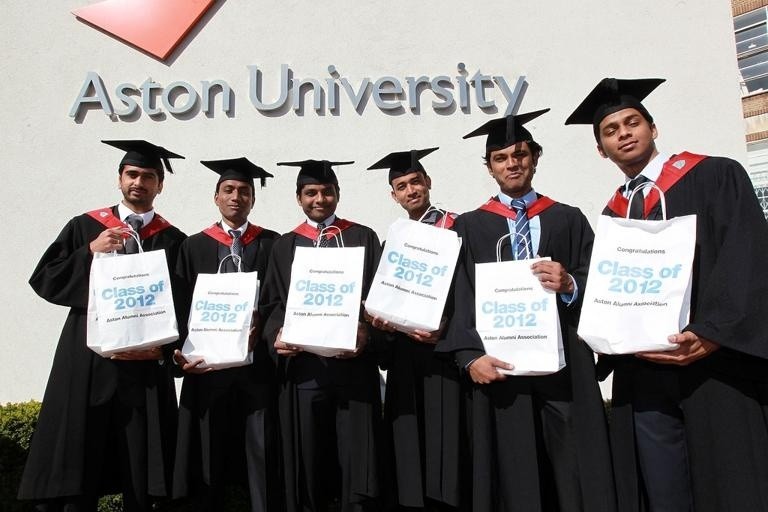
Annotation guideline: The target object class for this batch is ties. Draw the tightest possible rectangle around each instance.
[511,199,534,260]
[317,223,328,247]
[125,215,143,254]
[628,176,648,219]
[229,230,242,268]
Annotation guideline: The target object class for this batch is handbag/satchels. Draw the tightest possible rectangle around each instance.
[576,182,697,355]
[364,202,463,334]
[475,232,567,376]
[87,228,180,358]
[280,225,366,357]
[181,254,261,370]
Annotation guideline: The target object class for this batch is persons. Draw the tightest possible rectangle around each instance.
[435,109,617,512]
[565,78,767,512]
[15,139,186,511]
[163,156,282,512]
[362,147,469,511]
[265,160,381,512]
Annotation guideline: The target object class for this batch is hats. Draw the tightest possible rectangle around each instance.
[463,108,550,151]
[277,160,354,185]
[200,157,274,186]
[101,140,186,170]
[367,147,439,185]
[565,78,666,130]
